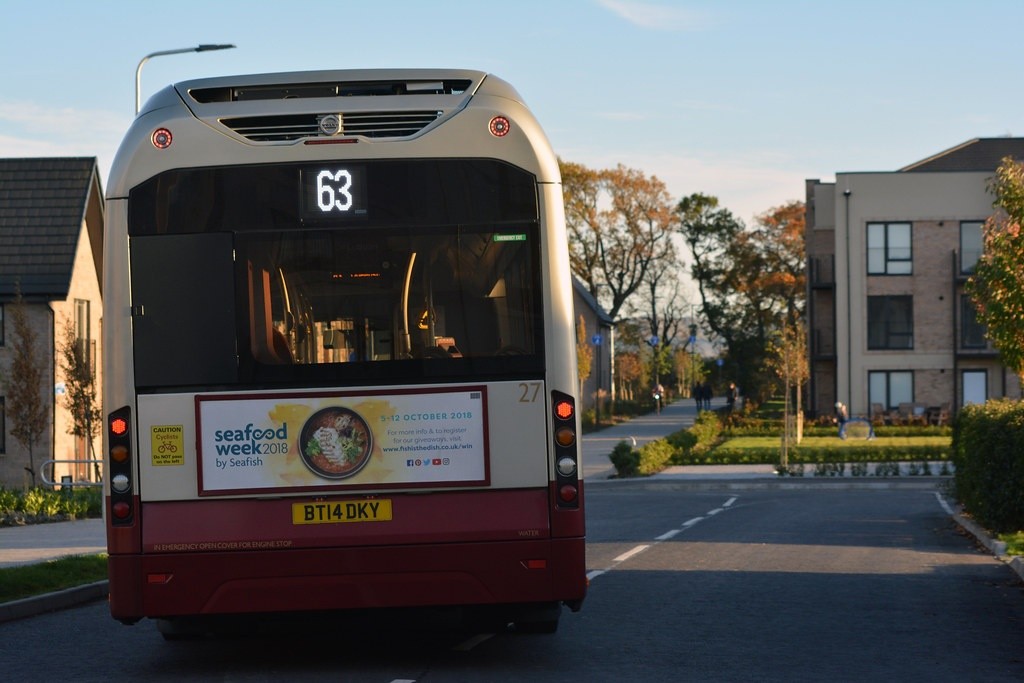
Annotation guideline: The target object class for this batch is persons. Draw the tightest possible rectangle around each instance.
[409,294,434,355]
[651,384,664,414]
[836,402,848,439]
[702,382,713,411]
[692,381,701,411]
[727,381,739,404]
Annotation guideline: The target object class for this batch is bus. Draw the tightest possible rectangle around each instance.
[97,66,589,640]
